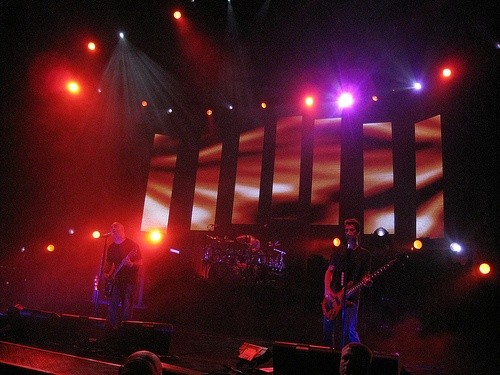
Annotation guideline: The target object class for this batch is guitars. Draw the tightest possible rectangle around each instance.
[322,256,398,321]
[104,246,138,298]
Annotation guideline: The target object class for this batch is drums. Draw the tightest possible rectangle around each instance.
[205,246,286,273]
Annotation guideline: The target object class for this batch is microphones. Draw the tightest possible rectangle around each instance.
[347,238,351,249]
[102,233,111,238]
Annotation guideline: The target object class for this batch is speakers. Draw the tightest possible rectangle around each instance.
[368,351,400,375]
[272,341,340,375]
[8,306,171,357]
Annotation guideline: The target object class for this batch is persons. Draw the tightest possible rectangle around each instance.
[324,219,370,351]
[120,350,163,375]
[339,342,372,375]
[104,222,142,321]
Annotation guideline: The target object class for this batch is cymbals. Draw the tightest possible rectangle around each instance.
[205,232,235,244]
